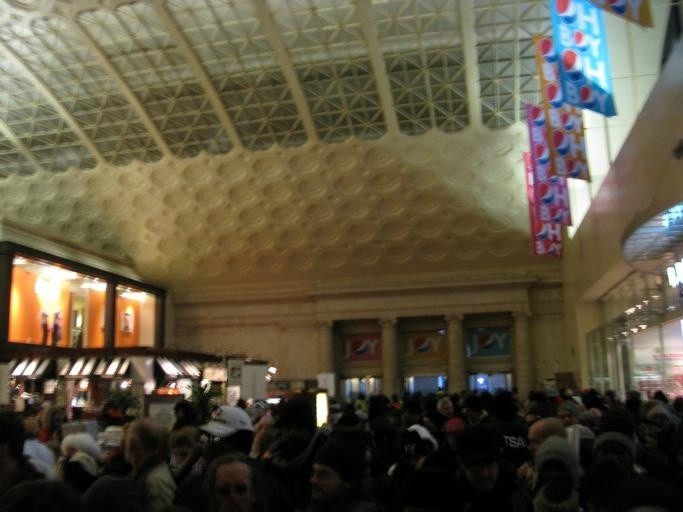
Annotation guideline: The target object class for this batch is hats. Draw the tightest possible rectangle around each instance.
[200,406,255,437]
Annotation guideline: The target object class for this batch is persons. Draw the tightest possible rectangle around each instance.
[1,383,682,510]
